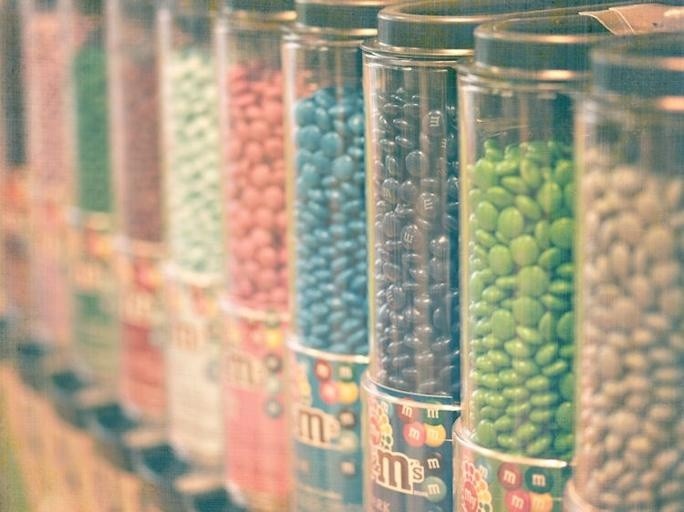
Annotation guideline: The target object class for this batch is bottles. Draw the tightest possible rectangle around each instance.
[21,0,67,350]
[214,1,294,511]
[106,1,162,424]
[0,317,251,512]
[452,15,613,511]
[564,34,682,512]
[361,1,569,511]
[156,0,219,462]
[284,1,398,511]
[65,0,108,378]
